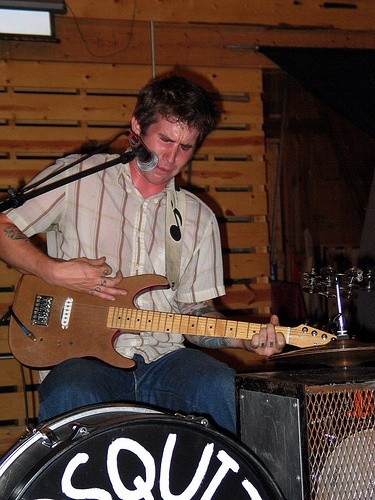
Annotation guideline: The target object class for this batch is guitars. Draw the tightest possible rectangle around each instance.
[7,272,338,371]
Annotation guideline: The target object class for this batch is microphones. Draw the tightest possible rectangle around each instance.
[126,131,158,171]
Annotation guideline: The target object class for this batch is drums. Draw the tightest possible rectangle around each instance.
[0,399,288,500]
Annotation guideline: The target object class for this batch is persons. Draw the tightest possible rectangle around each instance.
[0,77,287,444]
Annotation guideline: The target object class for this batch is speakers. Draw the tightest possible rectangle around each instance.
[231,368,374,499]
